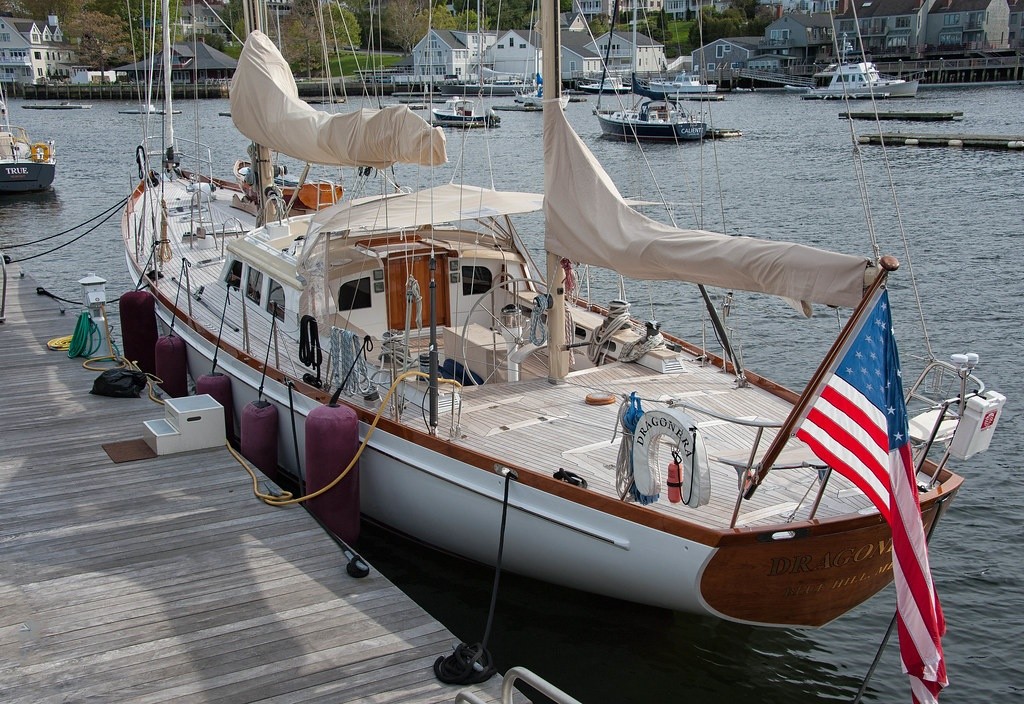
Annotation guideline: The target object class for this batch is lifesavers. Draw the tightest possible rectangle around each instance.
[632,408,711,508]
[33,143,50,163]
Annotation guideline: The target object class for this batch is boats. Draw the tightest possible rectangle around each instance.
[803,31,920,96]
[379,97,447,110]
[578,78,632,95]
[117,105,182,116]
[630,73,725,102]
[857,131,1024,154]
[21,101,93,110]
[300,96,346,107]
[0,81,57,191]
[430,95,501,128]
[118,1,1010,629]
[649,70,718,92]
[838,108,964,122]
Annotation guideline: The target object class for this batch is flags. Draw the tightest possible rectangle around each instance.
[796,288,949,704]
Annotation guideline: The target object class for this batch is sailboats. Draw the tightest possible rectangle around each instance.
[434,1,540,95]
[591,0,710,141]
[512,0,571,105]
